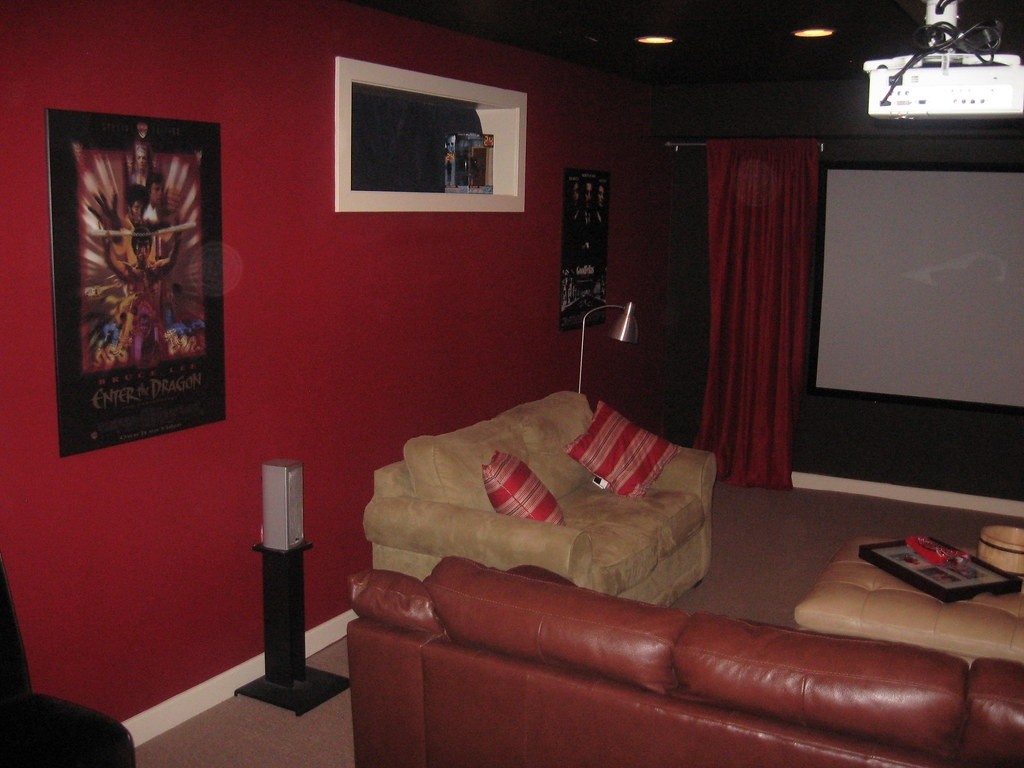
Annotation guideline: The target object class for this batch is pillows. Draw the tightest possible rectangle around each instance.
[483,450,567,528]
[563,401,679,498]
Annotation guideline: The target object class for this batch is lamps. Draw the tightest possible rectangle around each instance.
[578,301,639,395]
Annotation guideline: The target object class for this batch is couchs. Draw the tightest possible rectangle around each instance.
[363,392,717,609]
[346,555,1024,768]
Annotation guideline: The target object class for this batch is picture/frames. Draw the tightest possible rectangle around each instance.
[335,55,527,212]
[858,538,1021,605]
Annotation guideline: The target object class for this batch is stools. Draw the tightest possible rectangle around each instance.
[794,533,1024,671]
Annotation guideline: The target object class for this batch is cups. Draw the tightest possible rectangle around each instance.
[977,524,1024,575]
